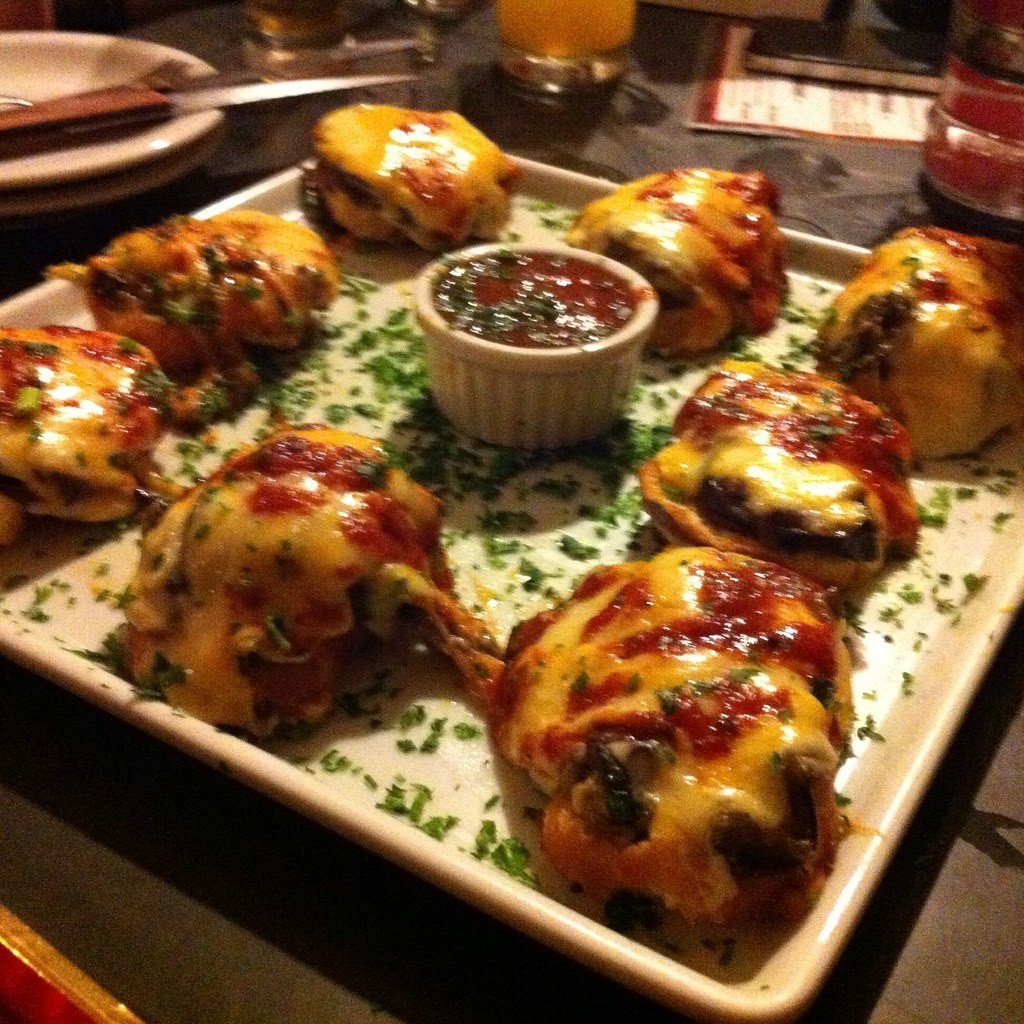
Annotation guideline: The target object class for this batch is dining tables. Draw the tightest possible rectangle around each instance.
[3,6,1023,1024]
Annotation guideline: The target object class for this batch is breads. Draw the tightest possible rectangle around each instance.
[0,101,1024,922]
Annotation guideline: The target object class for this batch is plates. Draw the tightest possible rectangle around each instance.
[2,28,221,183]
[0,128,1024,1024]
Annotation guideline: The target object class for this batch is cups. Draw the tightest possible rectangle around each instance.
[412,241,656,449]
[496,0,637,96]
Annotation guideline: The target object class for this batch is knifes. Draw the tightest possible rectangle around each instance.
[3,33,420,158]
